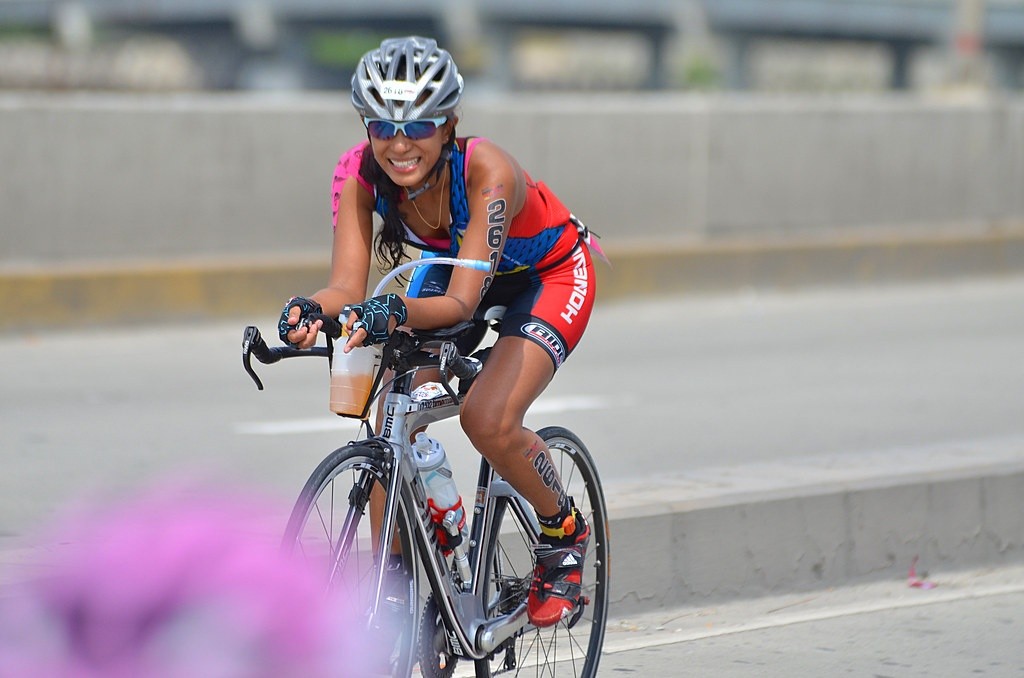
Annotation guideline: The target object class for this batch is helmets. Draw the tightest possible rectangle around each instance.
[1,481,390,678]
[351,36,464,122]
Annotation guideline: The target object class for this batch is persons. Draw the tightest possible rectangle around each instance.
[277,35,597,651]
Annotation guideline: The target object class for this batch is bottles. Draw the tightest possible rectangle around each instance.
[330,325,380,420]
[411,433,468,557]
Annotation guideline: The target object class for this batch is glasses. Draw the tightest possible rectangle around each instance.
[363,116,447,140]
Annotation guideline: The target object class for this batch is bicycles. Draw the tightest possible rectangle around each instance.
[242,300,614,677]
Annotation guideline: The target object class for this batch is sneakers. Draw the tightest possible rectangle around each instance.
[357,588,407,661]
[527,496,591,628]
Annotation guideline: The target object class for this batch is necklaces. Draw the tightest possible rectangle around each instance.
[402,155,448,231]
